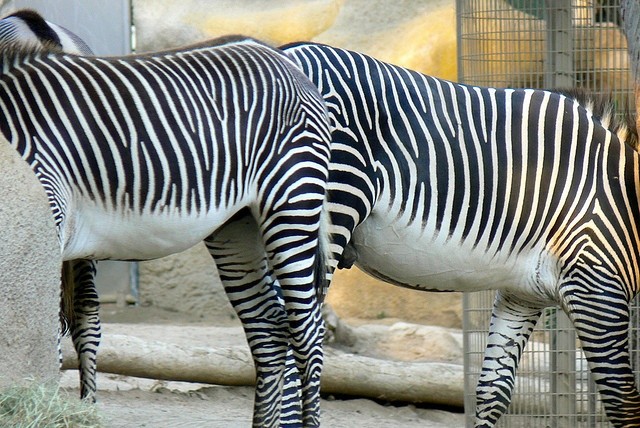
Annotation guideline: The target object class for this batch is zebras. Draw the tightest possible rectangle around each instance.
[0,9,101,405]
[0,35,333,428]
[274,41,640,428]
